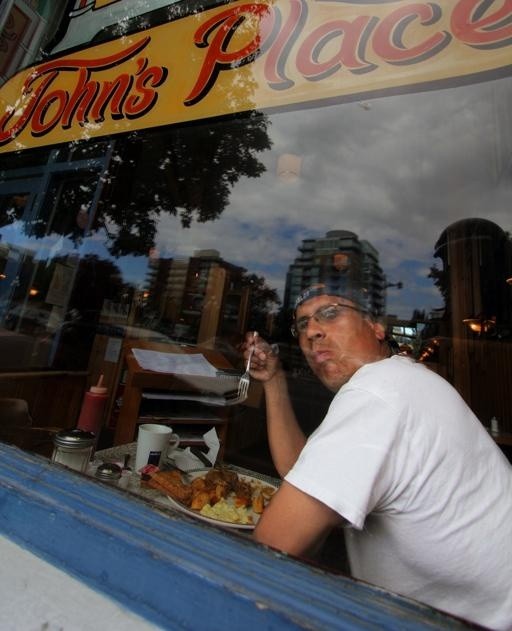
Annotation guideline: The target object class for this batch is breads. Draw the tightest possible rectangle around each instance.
[148,470,192,507]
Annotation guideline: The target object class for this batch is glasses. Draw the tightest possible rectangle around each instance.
[291,303,376,337]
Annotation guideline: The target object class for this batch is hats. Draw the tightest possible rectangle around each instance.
[290,275,378,314]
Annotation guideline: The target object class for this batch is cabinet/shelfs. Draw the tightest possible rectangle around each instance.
[106,338,238,462]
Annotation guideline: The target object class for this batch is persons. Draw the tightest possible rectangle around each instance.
[241,275,512,631]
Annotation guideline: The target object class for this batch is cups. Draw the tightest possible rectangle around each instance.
[135,424,181,478]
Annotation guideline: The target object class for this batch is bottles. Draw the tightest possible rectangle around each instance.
[77,374,110,442]
[51,428,97,476]
[88,458,133,490]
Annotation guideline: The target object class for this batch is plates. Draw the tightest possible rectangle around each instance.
[167,469,279,531]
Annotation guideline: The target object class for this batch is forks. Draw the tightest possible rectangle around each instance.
[235,331,260,399]
